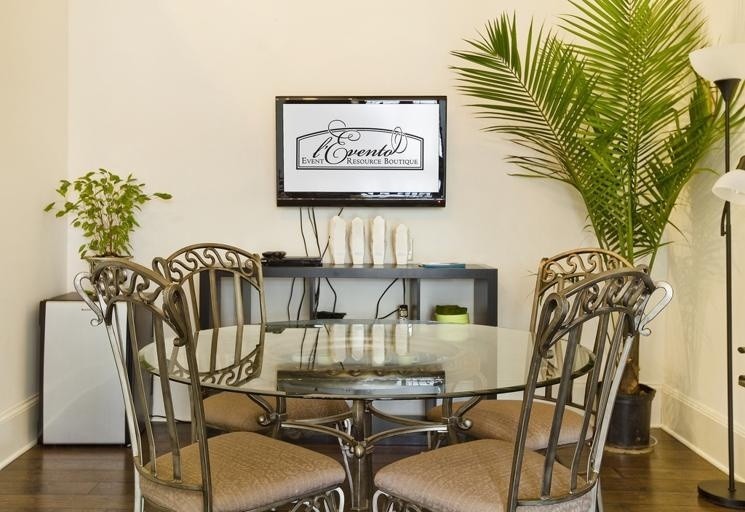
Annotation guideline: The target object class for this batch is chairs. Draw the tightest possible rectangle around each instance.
[151,244,354,512]
[372,265,673,512]
[426,247,633,465]
[73,258,346,512]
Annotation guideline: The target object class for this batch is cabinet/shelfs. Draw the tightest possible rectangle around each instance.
[41,291,153,445]
[199,263,498,445]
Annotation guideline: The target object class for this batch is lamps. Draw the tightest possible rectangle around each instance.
[689,45,745,510]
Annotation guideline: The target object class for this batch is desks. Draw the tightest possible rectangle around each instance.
[137,318,597,512]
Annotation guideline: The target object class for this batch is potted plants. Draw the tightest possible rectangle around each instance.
[450,0,745,454]
[43,168,172,300]
